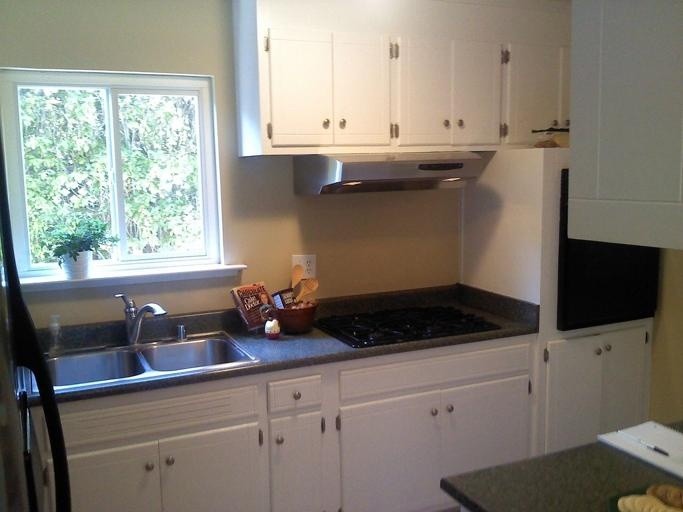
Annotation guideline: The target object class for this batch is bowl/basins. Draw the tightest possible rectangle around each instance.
[265,300,319,334]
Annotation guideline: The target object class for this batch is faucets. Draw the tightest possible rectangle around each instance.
[114,293,168,345]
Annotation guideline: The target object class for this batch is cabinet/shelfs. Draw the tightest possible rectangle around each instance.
[501,1,572,149]
[387,0,502,154]
[333,332,540,511]
[267,362,333,511]
[231,2,392,159]
[25,372,267,512]
[539,325,655,455]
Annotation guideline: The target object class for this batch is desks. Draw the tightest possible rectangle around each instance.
[440,420,682,511]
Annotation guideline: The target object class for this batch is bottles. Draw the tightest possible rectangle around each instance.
[47,313,66,360]
[265,317,279,338]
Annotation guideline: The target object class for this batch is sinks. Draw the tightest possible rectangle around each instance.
[140,330,261,378]
[30,343,147,398]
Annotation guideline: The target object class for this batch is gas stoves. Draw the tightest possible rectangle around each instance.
[313,304,506,352]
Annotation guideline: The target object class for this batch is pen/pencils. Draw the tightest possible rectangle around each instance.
[617,429,670,457]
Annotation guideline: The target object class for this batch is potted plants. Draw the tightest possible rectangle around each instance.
[40,213,118,280]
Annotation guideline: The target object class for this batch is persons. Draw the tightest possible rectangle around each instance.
[257,292,274,321]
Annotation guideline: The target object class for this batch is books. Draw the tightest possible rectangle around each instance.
[596,417,682,480]
[228,280,280,337]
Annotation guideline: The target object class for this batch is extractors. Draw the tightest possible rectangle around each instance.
[290,148,501,196]
